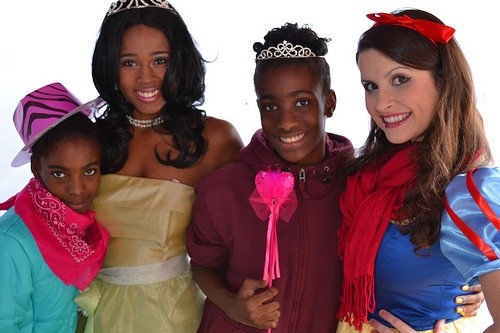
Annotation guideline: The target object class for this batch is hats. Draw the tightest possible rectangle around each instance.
[9,83,106,171]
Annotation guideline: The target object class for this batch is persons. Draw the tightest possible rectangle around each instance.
[335,10,500,333]
[186,23,484,333]
[75,0,244,333]
[0,81,110,333]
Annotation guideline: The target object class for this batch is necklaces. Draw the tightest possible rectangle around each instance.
[127,115,168,128]
